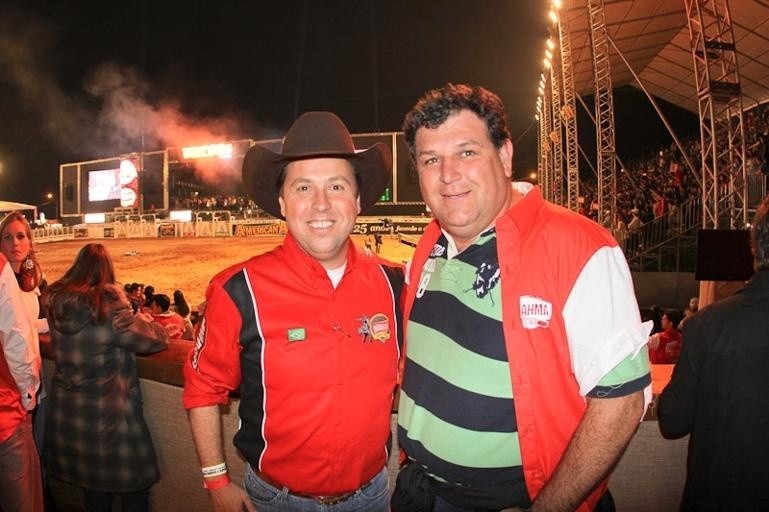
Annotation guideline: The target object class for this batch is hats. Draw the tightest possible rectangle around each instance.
[241,111,393,222]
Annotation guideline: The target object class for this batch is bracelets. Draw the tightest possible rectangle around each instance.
[201,462,231,491]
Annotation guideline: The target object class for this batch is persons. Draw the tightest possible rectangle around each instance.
[173,195,270,221]
[182,112,408,512]
[544,104,769,269]
[122,282,203,342]
[0,250,45,512]
[648,297,699,364]
[389,81,654,512]
[39,244,169,510]
[658,194,769,511]
[364,218,401,254]
[0,211,48,401]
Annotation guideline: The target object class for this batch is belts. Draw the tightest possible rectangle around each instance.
[251,464,372,506]
